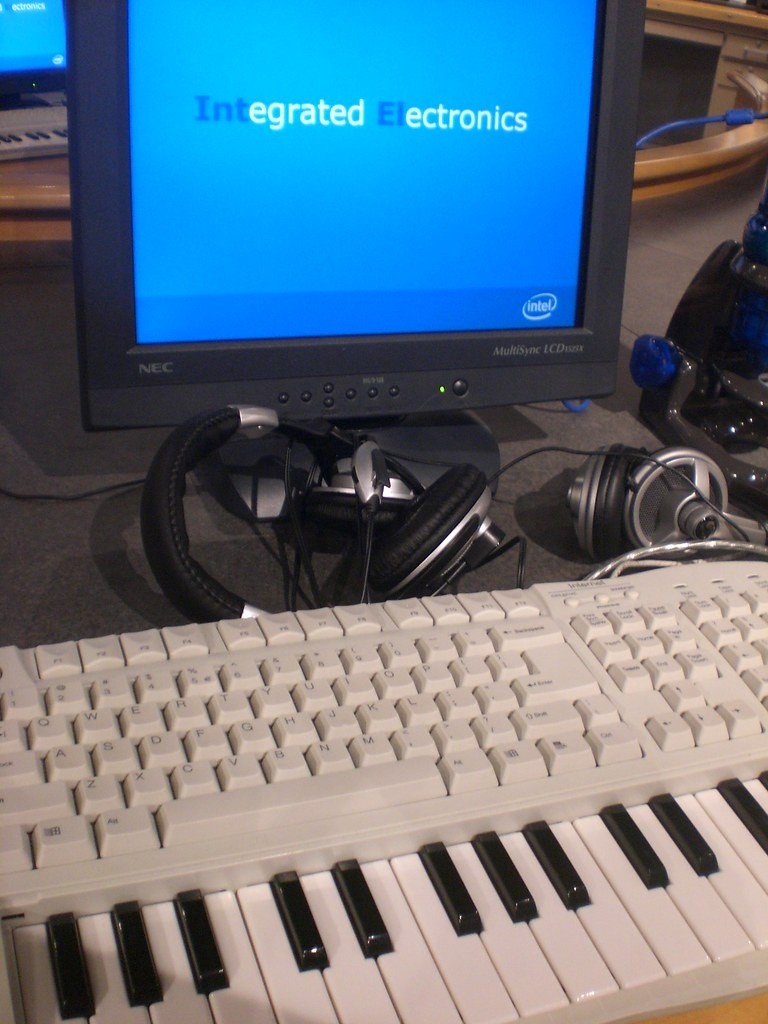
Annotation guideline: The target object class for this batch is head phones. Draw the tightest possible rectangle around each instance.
[141,403,506,621]
[564,442,768,566]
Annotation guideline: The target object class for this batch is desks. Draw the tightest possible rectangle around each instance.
[0,158,768,1024]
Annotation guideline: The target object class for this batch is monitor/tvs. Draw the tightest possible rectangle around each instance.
[0,0,646,520]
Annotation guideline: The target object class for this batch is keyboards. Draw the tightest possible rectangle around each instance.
[0,562,768,926]
